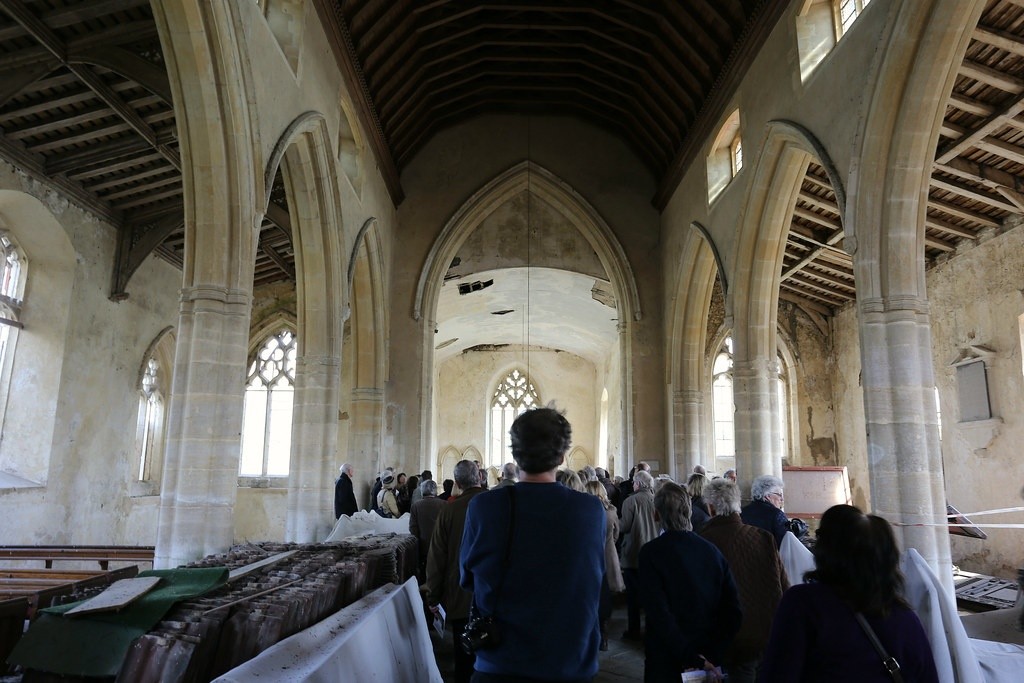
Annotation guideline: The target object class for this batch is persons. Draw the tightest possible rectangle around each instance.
[334,462,358,522]
[426,460,490,683]
[635,482,744,683]
[699,477,792,683]
[764,505,939,683]
[459,408,608,683]
[371,460,810,653]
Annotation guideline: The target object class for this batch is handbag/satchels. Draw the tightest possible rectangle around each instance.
[376,490,392,518]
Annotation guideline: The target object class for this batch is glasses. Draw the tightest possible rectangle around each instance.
[769,491,782,499]
[634,465,638,469]
[632,481,639,485]
[816,529,819,538]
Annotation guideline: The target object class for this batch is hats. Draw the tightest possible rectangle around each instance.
[382,475,396,487]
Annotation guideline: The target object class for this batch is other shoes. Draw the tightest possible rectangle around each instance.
[599,638,608,651]
[622,631,642,641]
